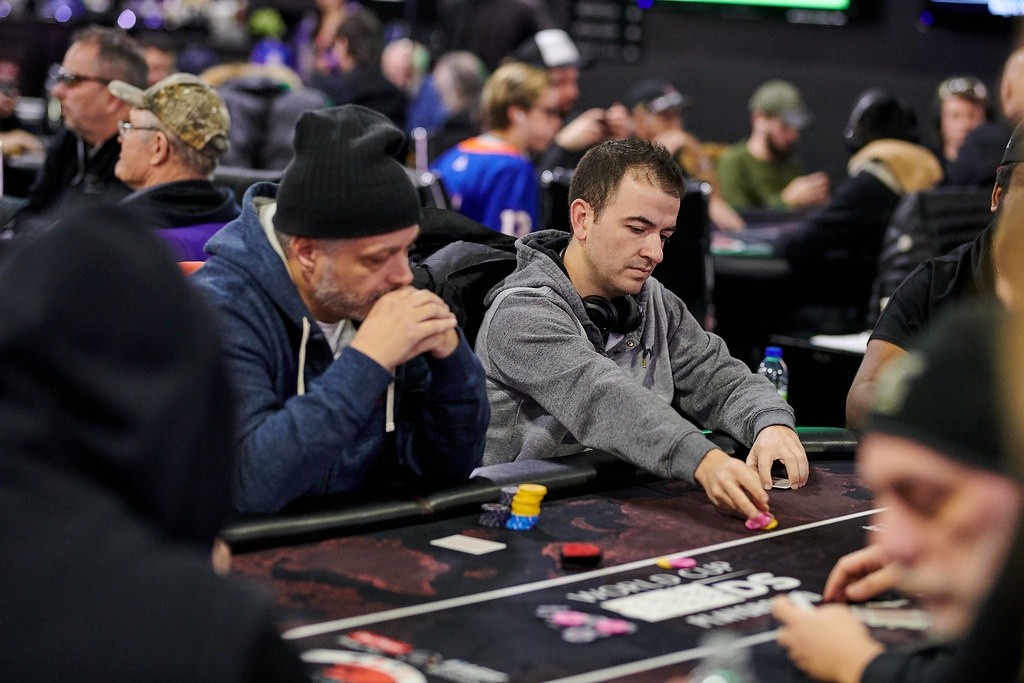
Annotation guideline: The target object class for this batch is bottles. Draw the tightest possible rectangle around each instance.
[757,346,789,402]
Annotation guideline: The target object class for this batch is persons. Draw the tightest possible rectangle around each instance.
[715,80,829,215]
[506,30,636,166]
[0,202,335,683]
[929,74,997,166]
[868,50,1024,331]
[0,0,562,261]
[769,291,1024,683]
[474,136,809,519]
[715,88,945,364]
[188,108,492,524]
[620,80,747,229]
[846,118,1024,440]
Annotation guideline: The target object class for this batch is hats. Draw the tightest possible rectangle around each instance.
[512,30,599,73]
[620,77,689,118]
[1000,118,1023,165]
[749,78,813,129]
[107,73,230,158]
[844,90,919,148]
[867,299,1024,484]
[271,105,420,234]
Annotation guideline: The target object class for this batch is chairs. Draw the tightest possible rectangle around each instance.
[540,171,714,332]
[770,189,996,356]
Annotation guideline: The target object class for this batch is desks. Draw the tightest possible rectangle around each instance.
[222,426,921,681]
[709,226,798,276]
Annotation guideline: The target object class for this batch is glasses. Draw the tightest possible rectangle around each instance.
[115,121,179,154]
[48,62,112,86]
[647,90,684,113]
[0,80,19,97]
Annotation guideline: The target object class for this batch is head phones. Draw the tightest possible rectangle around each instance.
[581,294,644,334]
